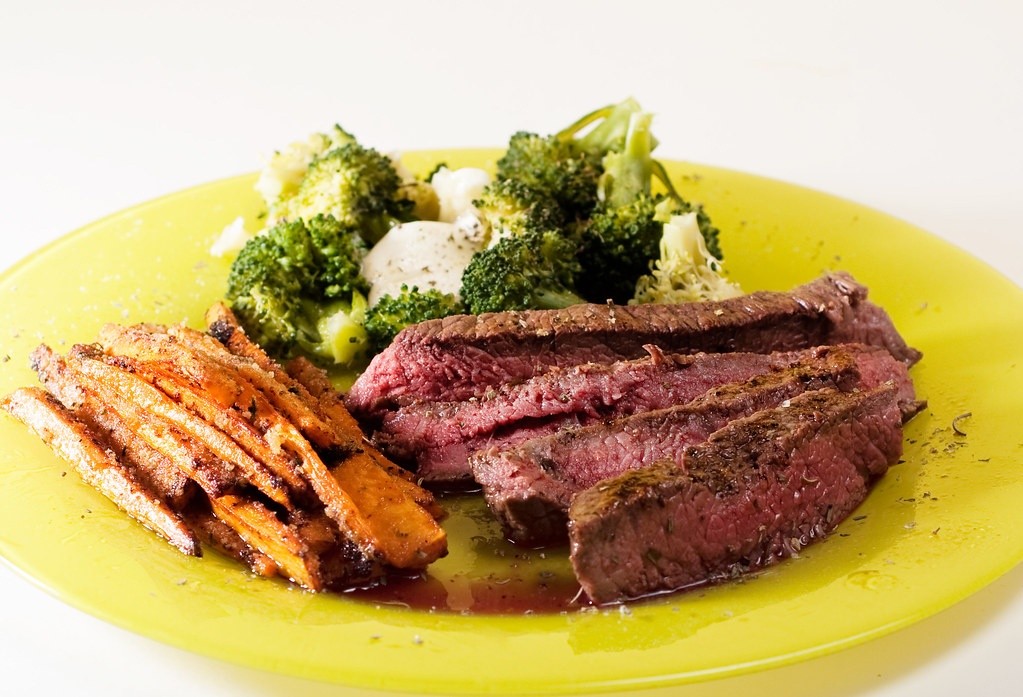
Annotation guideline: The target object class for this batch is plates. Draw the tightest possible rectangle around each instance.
[0,150,1021,697]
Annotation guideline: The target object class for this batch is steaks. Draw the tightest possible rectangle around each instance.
[347,270,926,606]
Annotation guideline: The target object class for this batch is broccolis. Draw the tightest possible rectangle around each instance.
[223,98,744,367]
